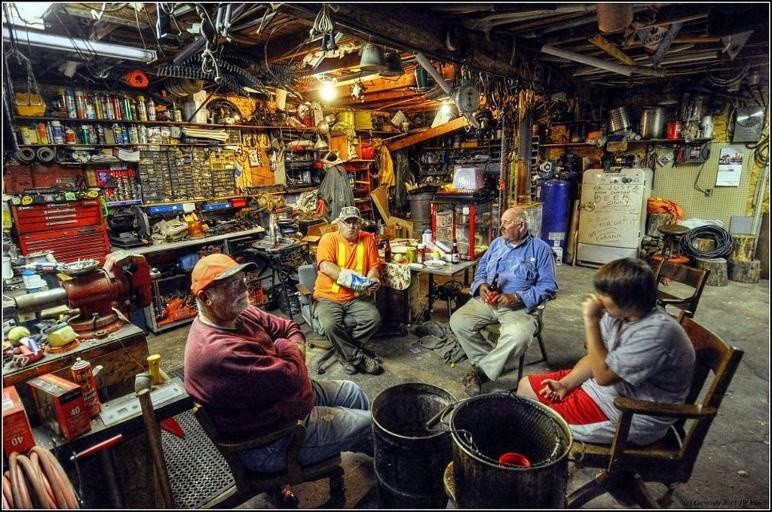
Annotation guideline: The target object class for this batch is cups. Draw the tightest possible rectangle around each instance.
[2,256,13,282]
[174,111,182,122]
[188,220,203,237]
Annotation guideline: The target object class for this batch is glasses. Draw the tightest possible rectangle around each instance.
[196,274,247,293]
[339,219,362,227]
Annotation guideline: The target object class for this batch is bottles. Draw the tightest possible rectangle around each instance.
[383,236,392,262]
[20,119,147,147]
[407,244,427,264]
[57,87,156,121]
[432,249,441,260]
[451,242,460,264]
[22,269,39,290]
[71,355,103,419]
[421,229,432,243]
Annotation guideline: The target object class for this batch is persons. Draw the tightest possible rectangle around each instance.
[515,259,696,445]
[184,251,375,473]
[313,207,382,375]
[448,207,559,398]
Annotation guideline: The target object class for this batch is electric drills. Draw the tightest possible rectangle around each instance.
[12,261,65,274]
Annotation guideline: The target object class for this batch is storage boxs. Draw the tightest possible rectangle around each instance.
[1,384,37,461]
[27,373,93,443]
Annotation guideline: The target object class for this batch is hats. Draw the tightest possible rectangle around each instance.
[190,252,257,296]
[336,206,364,221]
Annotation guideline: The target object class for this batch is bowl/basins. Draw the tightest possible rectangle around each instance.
[23,347,44,362]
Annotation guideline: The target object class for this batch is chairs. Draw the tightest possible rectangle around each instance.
[582,257,710,349]
[566,308,745,508]
[192,402,347,509]
[482,294,555,393]
[297,264,382,374]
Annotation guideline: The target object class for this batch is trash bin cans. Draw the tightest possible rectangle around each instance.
[406,192,435,233]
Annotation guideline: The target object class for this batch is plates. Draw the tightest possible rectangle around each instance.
[390,246,415,253]
[422,259,446,269]
[56,260,100,274]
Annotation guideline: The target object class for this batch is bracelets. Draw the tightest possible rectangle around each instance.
[514,293,521,301]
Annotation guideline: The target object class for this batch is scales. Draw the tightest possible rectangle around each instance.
[454,70,480,133]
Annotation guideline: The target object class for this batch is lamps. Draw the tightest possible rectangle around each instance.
[3,3,159,65]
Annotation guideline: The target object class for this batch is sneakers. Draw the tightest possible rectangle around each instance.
[343,350,379,375]
[461,364,483,396]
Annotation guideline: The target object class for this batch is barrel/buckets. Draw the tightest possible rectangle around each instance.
[355,111,373,130]
[390,110,407,126]
[370,381,457,510]
[434,209,454,242]
[647,213,673,238]
[639,107,665,140]
[608,106,632,133]
[448,393,573,509]
[184,101,208,125]
[691,235,715,253]
[727,232,757,261]
[336,112,355,130]
[407,192,432,220]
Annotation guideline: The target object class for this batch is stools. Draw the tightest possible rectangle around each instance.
[656,223,691,257]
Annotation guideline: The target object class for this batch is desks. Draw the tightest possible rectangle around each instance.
[376,259,482,321]
[2,265,154,429]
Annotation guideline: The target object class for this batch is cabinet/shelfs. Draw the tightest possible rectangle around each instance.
[332,128,377,221]
[108,224,270,334]
[417,134,541,180]
[13,115,321,209]
